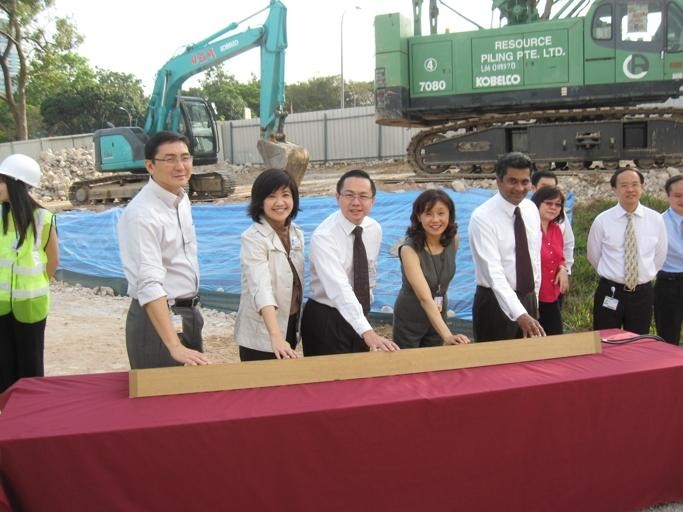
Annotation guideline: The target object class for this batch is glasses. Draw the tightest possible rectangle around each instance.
[154,155,193,164]
[542,200,561,207]
[341,192,375,200]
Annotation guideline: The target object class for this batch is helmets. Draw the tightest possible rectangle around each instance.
[0,153,41,189]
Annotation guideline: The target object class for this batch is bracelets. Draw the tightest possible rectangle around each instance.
[557,268,568,273]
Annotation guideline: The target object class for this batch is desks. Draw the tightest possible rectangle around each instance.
[1,326,682,509]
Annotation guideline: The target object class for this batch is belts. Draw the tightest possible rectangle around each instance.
[609,280,640,293]
[177,295,200,307]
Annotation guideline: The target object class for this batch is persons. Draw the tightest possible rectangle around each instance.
[528,187,570,336]
[116,130,211,367]
[652,174,682,347]
[299,167,401,354]
[0,153,60,391]
[231,168,306,361]
[528,170,574,276]
[467,151,549,340]
[391,185,472,347]
[585,167,667,336]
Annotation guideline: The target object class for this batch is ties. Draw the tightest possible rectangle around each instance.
[352,226,370,316]
[514,207,533,297]
[624,213,638,290]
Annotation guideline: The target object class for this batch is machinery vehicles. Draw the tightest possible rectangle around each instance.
[68,1,308,207]
[371,1,682,181]
